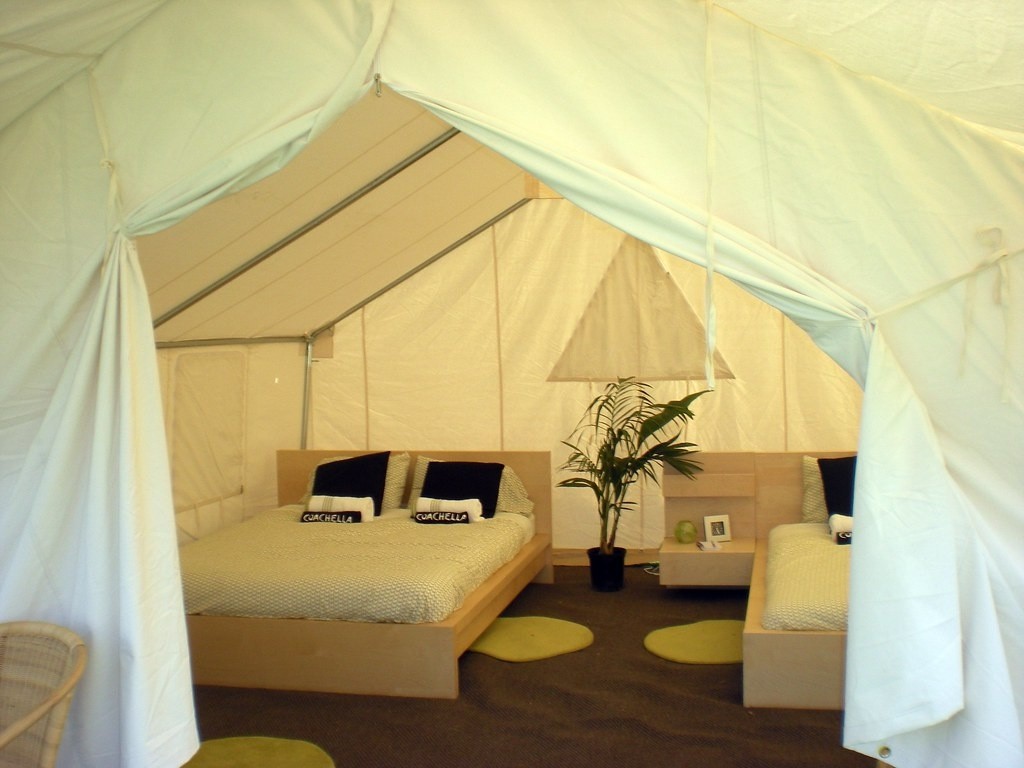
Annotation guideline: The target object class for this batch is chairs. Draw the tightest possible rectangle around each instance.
[0,620,87,767]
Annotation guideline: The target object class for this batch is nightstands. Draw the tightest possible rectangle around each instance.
[659,453,754,584]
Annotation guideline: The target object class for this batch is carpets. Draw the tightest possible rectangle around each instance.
[469,614,594,662]
[645,619,745,665]
[181,734,337,767]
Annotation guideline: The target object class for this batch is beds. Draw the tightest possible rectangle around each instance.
[178,449,553,699]
[742,451,857,707]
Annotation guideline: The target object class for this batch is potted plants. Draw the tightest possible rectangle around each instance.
[553,375,716,591]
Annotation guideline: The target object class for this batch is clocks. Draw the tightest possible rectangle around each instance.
[704,514,731,542]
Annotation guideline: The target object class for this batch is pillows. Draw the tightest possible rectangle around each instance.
[817,456,857,517]
[301,454,411,510]
[312,449,389,516]
[802,455,829,523]
[406,455,534,514]
[421,460,506,518]
